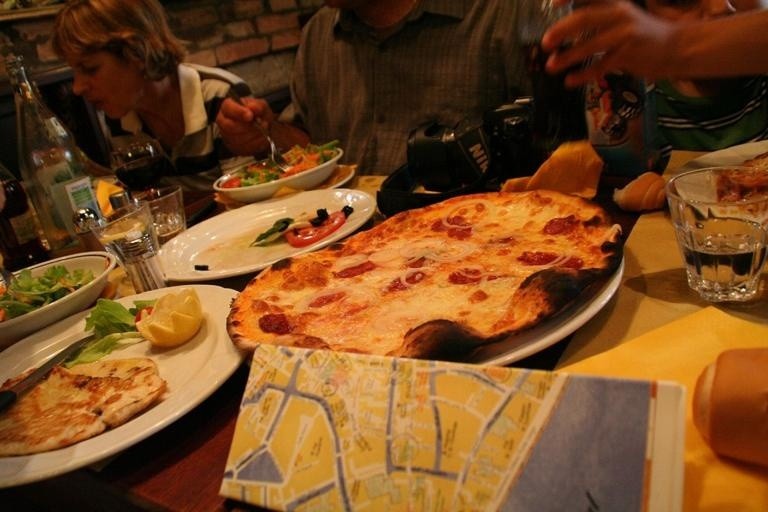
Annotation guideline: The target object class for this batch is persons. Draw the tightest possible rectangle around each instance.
[29,1,254,177]
[540,1,768,176]
[215,0,577,177]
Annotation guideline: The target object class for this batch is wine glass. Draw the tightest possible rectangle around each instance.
[110,139,160,192]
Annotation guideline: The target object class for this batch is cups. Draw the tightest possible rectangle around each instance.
[665,166,766,306]
[141,185,187,243]
[93,204,159,278]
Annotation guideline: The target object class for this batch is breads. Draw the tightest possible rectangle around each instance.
[692,350,768,469]
[526,140,668,208]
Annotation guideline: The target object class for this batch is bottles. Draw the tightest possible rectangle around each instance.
[584,52,647,174]
[0,167,47,263]
[5,56,134,252]
[119,233,166,293]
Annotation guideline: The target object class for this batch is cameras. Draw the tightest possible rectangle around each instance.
[406,39,587,192]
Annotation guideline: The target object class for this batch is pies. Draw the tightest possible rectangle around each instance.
[0,354,166,457]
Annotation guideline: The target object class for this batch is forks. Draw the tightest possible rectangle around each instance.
[225,89,293,172]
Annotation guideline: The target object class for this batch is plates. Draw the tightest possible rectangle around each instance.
[208,164,357,203]
[1,252,116,339]
[468,256,632,367]
[675,141,767,168]
[214,146,341,199]
[158,190,375,287]
[0,285,246,484]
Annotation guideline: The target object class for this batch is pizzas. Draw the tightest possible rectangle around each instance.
[225,185,623,363]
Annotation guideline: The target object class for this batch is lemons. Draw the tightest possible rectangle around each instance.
[137,288,203,345]
